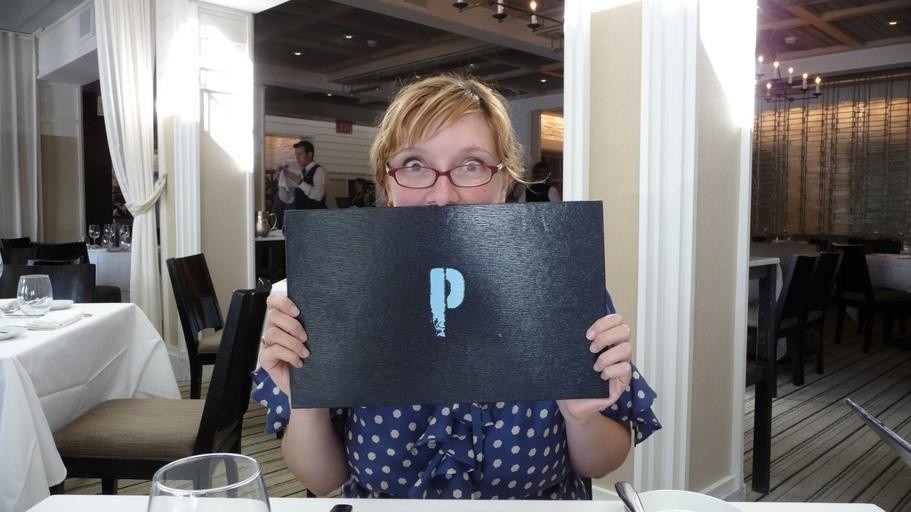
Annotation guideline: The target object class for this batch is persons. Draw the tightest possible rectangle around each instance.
[527,160,561,202]
[281,140,328,210]
[253,73,666,502]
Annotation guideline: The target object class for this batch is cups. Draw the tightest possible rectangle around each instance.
[145,450,271,512]
[17,273,54,316]
[256,210,278,237]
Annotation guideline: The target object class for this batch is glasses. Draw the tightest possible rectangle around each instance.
[383,160,506,190]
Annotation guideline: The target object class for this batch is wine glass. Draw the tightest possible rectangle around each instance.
[89,223,131,251]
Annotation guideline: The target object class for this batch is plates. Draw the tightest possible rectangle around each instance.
[618,488,746,511]
[1,299,72,343]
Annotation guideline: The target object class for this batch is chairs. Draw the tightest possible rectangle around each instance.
[746,230,910,397]
[165,253,224,399]
[0,234,161,303]
[49,277,272,497]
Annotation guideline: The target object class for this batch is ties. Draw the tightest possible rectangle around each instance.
[301,168,307,178]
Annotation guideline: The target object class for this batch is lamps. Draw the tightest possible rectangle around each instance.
[452,0,542,34]
[755,15,823,105]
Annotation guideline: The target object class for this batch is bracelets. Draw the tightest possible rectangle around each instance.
[299,180,303,185]
